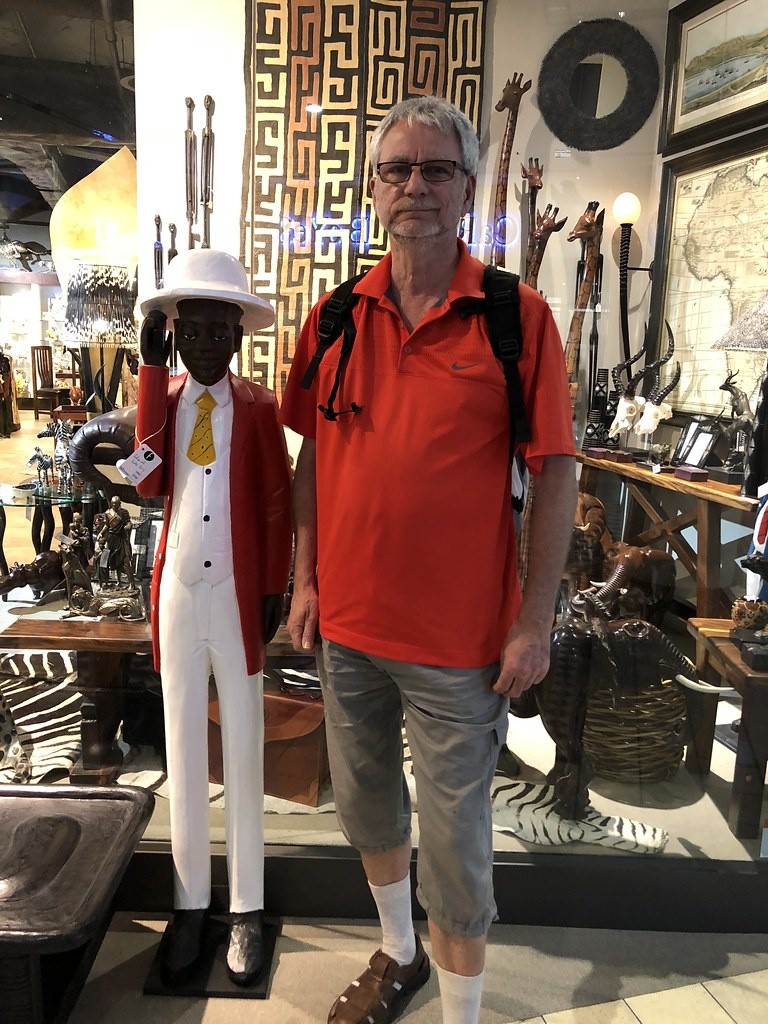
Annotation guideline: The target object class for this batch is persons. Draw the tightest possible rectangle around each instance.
[134,250,291,988]
[97,496,136,591]
[276,93,578,1024]
[63,512,106,611]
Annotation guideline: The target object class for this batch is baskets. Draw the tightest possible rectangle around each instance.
[533,619,697,785]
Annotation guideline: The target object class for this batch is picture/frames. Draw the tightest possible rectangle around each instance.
[656,0,768,158]
[641,127,768,431]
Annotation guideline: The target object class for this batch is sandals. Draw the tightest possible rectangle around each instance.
[327,929,430,1024]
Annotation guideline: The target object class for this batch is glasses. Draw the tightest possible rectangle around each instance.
[375,159,469,183]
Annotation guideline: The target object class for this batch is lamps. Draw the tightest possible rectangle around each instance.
[613,192,641,384]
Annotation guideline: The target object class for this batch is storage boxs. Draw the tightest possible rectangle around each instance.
[208,689,330,808]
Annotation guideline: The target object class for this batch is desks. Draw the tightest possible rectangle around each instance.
[576,449,768,840]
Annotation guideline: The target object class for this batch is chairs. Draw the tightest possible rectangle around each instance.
[31,346,69,420]
